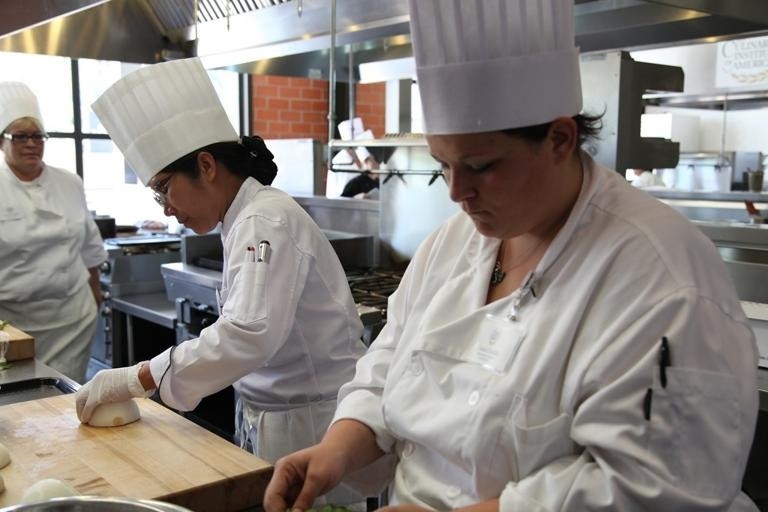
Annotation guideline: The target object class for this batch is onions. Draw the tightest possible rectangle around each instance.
[0,441,12,469]
[87,399,141,428]
[18,479,78,505]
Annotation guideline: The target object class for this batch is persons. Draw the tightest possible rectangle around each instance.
[0,118,111,387]
[262,103,768,510]
[73,136,399,511]
[339,157,380,200]
[629,169,669,189]
[325,148,362,199]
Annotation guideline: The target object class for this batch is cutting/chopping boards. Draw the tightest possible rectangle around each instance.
[1,378,275,512]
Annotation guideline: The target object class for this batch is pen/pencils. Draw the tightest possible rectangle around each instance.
[246,240,270,262]
[659,337,670,388]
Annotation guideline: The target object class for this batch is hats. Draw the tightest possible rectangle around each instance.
[338,116,377,164]
[406,2,582,137]
[91,56,240,189]
[0,82,45,134]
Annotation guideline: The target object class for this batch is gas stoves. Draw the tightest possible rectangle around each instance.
[336,252,414,323]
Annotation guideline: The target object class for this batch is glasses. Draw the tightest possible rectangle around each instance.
[2,131,50,145]
[150,174,174,205]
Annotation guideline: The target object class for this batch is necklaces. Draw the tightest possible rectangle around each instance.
[489,221,565,288]
